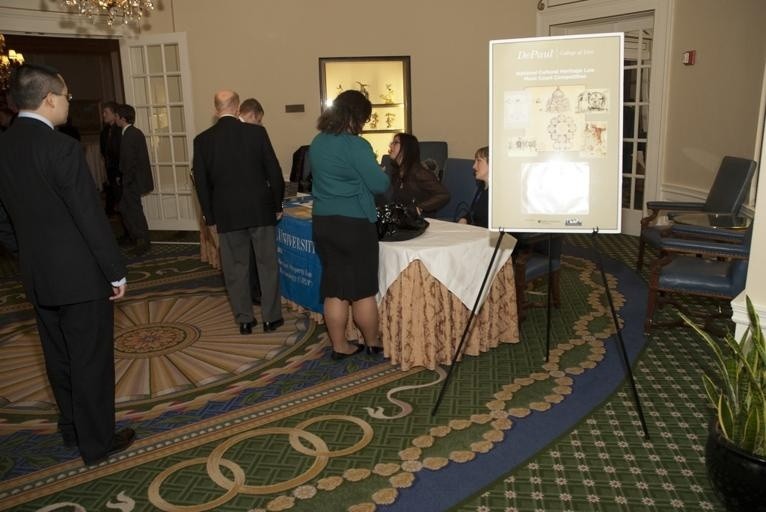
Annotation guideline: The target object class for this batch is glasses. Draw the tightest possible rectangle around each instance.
[391,140,401,145]
[41,91,74,103]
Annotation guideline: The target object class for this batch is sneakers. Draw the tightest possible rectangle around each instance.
[366,342,385,357]
[84,426,138,466]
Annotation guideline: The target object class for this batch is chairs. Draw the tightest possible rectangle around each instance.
[417,139,449,174]
[514,221,567,327]
[643,218,755,330]
[636,154,760,274]
[438,156,480,224]
[287,144,316,199]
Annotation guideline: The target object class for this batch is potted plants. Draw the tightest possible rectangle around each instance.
[669,294,766,511]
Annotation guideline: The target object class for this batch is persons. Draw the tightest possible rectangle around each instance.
[113,102,154,256]
[234,98,264,307]
[191,88,287,335]
[299,90,391,361]
[374,132,451,221]
[97,101,122,218]
[454,145,522,263]
[0,90,18,132]
[0,62,139,467]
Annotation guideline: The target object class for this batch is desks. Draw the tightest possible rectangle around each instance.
[665,209,754,250]
[190,188,517,368]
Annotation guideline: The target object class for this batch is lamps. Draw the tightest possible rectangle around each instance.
[48,0,158,34]
[0,32,24,92]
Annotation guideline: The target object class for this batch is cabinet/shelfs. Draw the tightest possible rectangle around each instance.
[315,54,412,168]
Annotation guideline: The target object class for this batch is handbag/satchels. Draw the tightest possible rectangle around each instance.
[375,198,432,240]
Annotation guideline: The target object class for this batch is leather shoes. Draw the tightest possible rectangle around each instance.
[261,316,286,333]
[239,317,257,335]
[329,343,366,361]
